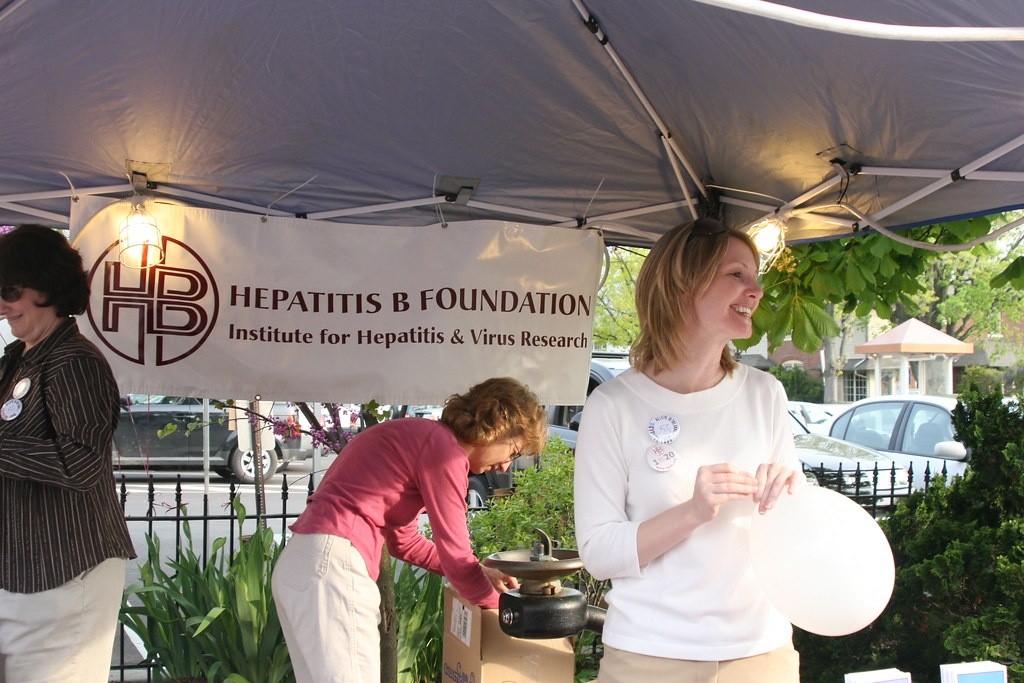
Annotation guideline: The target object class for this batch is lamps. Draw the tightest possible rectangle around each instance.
[119,194,165,269]
[744,214,790,277]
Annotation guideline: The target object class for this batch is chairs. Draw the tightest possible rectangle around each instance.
[908,422,942,457]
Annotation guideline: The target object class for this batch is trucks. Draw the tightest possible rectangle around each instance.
[462,350,635,513]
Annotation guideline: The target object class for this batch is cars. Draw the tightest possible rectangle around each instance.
[272,400,326,475]
[789,391,1024,509]
[109,393,278,484]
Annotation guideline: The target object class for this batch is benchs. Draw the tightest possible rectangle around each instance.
[850,415,892,449]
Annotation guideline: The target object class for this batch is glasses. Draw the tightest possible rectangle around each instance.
[0,285,26,303]
[685,215,726,244]
[510,433,521,460]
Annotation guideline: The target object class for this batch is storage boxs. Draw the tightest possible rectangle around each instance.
[442,582,576,683]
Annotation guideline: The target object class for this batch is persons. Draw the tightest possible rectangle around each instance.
[272,378,548,683]
[0,220,137,683]
[573,218,808,683]
